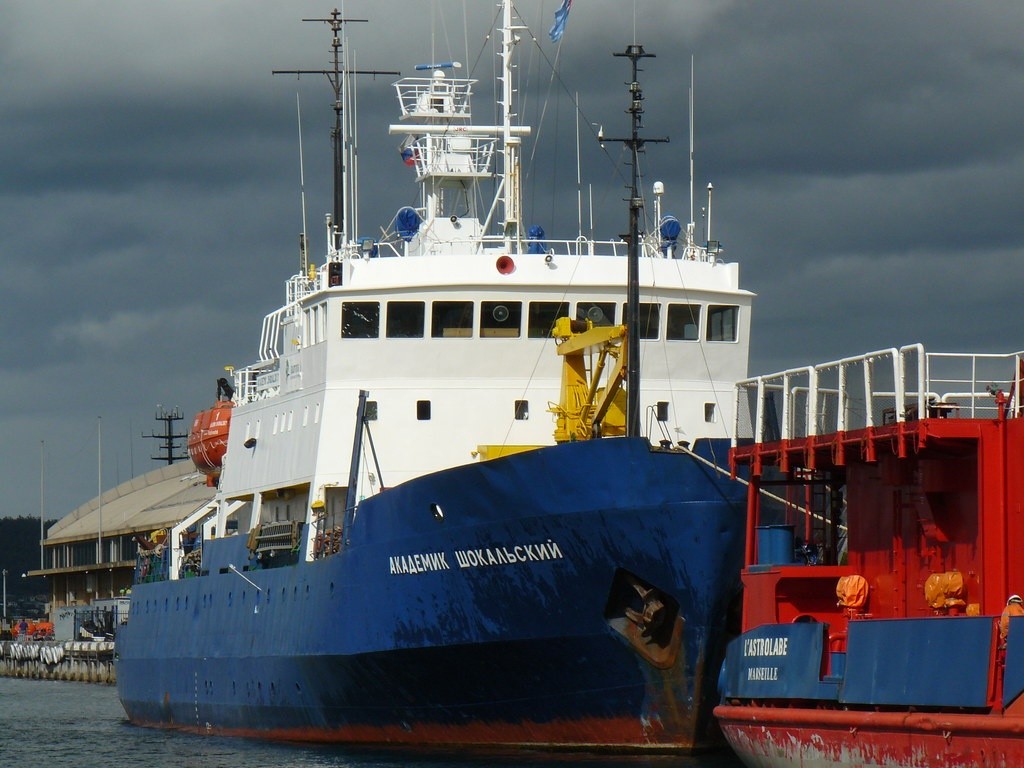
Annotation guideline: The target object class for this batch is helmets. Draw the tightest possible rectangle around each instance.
[1007,595,1022,605]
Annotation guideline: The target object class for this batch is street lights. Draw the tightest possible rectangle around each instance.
[3,568,8,640]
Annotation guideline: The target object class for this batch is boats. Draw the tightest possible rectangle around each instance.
[711,342,1023,768]
[112,0,838,768]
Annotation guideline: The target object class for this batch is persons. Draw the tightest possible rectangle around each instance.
[20,617,28,641]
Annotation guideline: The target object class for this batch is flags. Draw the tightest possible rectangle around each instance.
[548,0,571,41]
[399,137,420,166]
